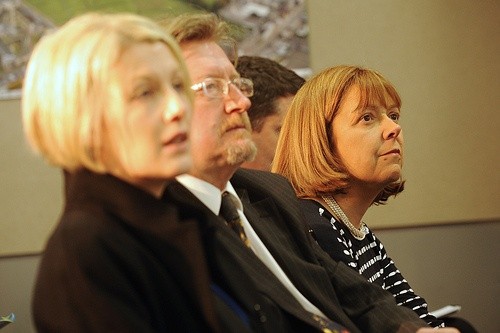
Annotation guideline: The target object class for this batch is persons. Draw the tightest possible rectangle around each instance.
[19,10,480,333]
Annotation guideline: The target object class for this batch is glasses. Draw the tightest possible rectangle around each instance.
[191,78,254,98]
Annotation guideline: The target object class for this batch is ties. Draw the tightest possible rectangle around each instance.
[218,191,350,332]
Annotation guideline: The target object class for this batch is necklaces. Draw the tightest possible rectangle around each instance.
[320,191,365,237]
